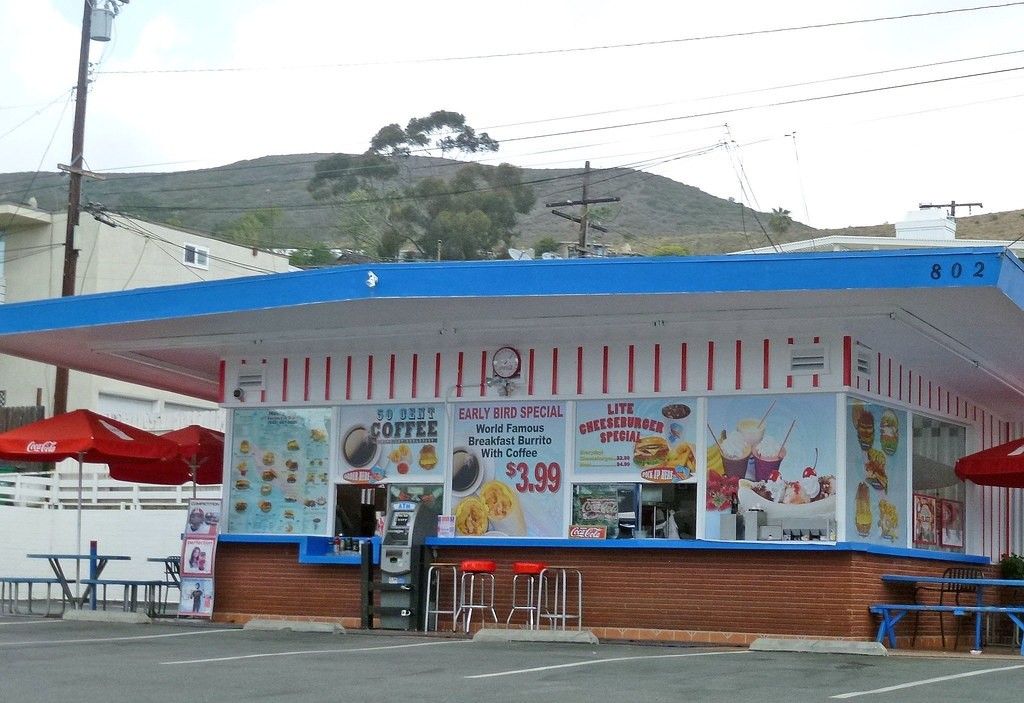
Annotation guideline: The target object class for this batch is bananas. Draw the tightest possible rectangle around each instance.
[706,429,729,475]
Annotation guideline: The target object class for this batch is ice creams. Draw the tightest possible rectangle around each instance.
[749,446,836,504]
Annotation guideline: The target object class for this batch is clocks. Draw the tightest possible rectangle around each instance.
[490,347,521,377]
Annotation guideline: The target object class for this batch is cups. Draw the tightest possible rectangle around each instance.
[855,498,872,536]
[880,514,895,539]
[720,442,752,480]
[752,440,786,482]
[736,419,766,447]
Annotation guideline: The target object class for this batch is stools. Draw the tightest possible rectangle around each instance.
[423,560,582,635]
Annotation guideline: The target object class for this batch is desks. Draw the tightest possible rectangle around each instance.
[146,557,182,614]
[883,573,1023,653]
[27,554,132,609]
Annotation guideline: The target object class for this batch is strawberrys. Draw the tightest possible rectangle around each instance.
[705,470,740,511]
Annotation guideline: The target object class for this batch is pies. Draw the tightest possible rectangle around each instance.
[454,482,526,537]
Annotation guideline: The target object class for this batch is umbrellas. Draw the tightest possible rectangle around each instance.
[0,409,159,609]
[912,453,958,491]
[109,425,225,499]
[954,437,1024,559]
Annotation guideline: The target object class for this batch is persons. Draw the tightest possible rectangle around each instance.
[189,547,206,571]
[190,583,211,613]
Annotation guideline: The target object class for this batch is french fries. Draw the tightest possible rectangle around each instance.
[663,444,695,472]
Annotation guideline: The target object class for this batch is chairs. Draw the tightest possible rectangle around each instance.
[910,566,983,649]
[163,554,183,613]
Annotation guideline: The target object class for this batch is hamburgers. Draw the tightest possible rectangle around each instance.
[236,479,251,490]
[632,436,670,468]
[858,413,875,445]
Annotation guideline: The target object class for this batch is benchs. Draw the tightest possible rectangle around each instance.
[0,580,181,618]
[869,605,1024,656]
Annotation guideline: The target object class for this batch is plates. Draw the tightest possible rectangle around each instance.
[737,479,835,517]
[451,445,484,498]
[660,403,692,421]
[339,422,381,471]
